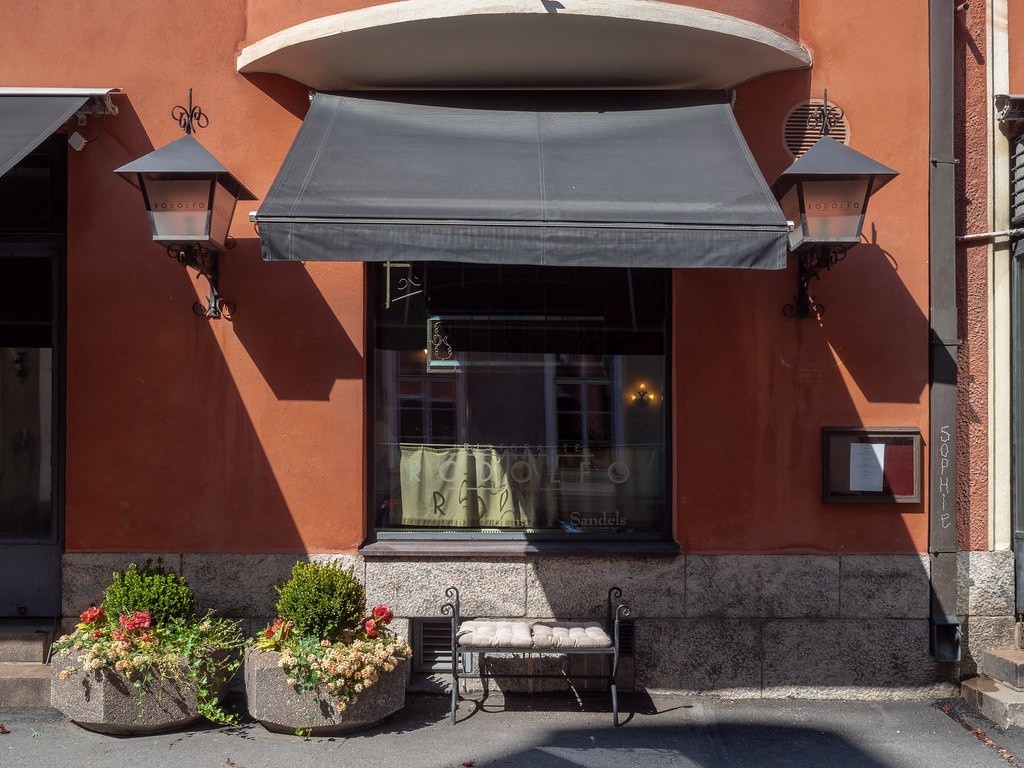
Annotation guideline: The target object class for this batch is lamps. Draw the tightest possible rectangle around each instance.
[770,87,900,317]
[629,382,657,407]
[113,89,259,320]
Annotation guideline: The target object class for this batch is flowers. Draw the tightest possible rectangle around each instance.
[52,607,162,679]
[264,605,413,712]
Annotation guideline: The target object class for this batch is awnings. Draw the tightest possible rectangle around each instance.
[1,93,95,178]
[248,86,795,272]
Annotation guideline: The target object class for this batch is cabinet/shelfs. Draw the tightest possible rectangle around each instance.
[441,585,631,727]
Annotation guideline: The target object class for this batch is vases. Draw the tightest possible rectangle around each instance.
[243,638,408,735]
[49,645,230,734]
